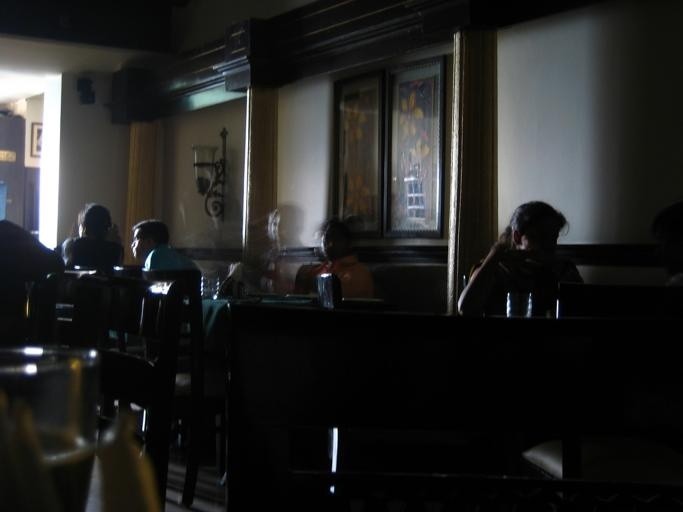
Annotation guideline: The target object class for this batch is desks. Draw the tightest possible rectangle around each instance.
[202,293,387,341]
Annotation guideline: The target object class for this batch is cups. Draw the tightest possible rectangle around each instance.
[506,291,533,319]
[1,346,100,511]
[200,275,220,300]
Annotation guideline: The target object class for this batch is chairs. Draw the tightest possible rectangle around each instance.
[172,242,683,317]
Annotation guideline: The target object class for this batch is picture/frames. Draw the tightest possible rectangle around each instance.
[332,68,381,240]
[383,53,447,240]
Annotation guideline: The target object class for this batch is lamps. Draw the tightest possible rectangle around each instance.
[190,127,229,221]
